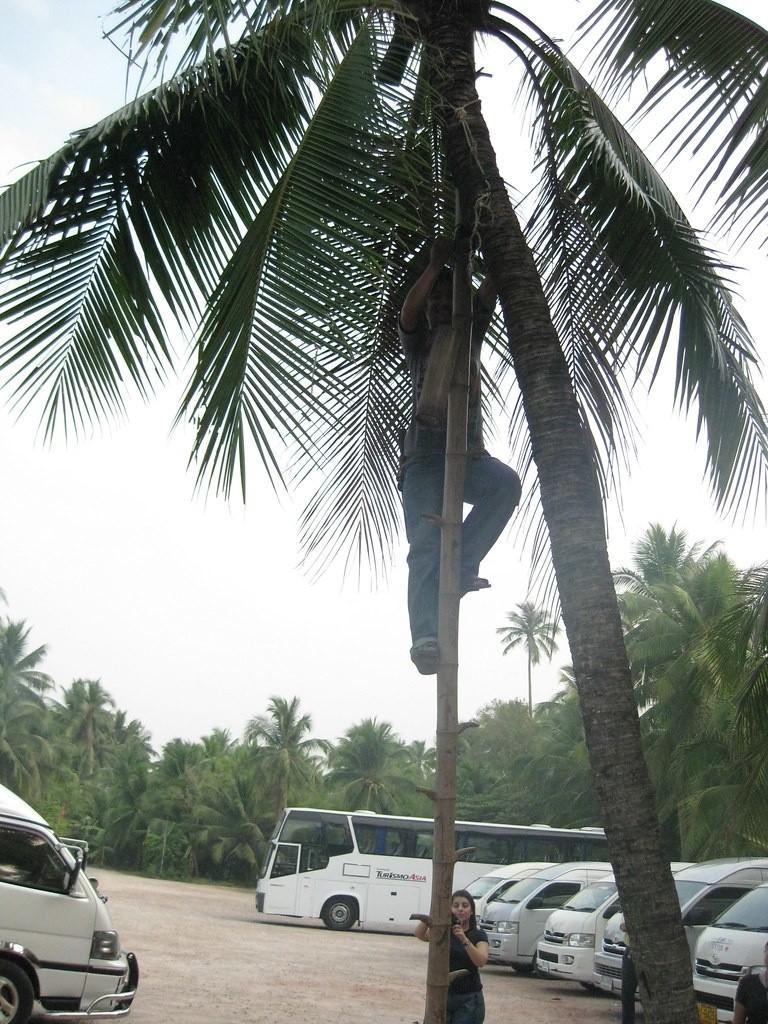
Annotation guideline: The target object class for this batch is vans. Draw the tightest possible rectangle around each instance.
[535,861,696,991]
[479,861,612,977]
[693,881,768,1024]
[0,780,139,1024]
[591,854,768,1003]
[460,862,559,926]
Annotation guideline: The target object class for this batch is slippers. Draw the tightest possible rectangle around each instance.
[411,643,439,675]
[463,578,491,588]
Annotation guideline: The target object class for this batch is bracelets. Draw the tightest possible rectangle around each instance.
[463,940,470,946]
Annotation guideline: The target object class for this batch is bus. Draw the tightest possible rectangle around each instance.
[254,806,613,931]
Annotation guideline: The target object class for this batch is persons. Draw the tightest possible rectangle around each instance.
[399,232,523,676]
[415,890,489,1024]
[733,942,768,1024]
[620,913,636,1024]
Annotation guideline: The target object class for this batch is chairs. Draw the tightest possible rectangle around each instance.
[416,846,427,857]
[389,844,404,857]
[500,856,509,863]
[361,838,374,853]
[544,851,614,863]
